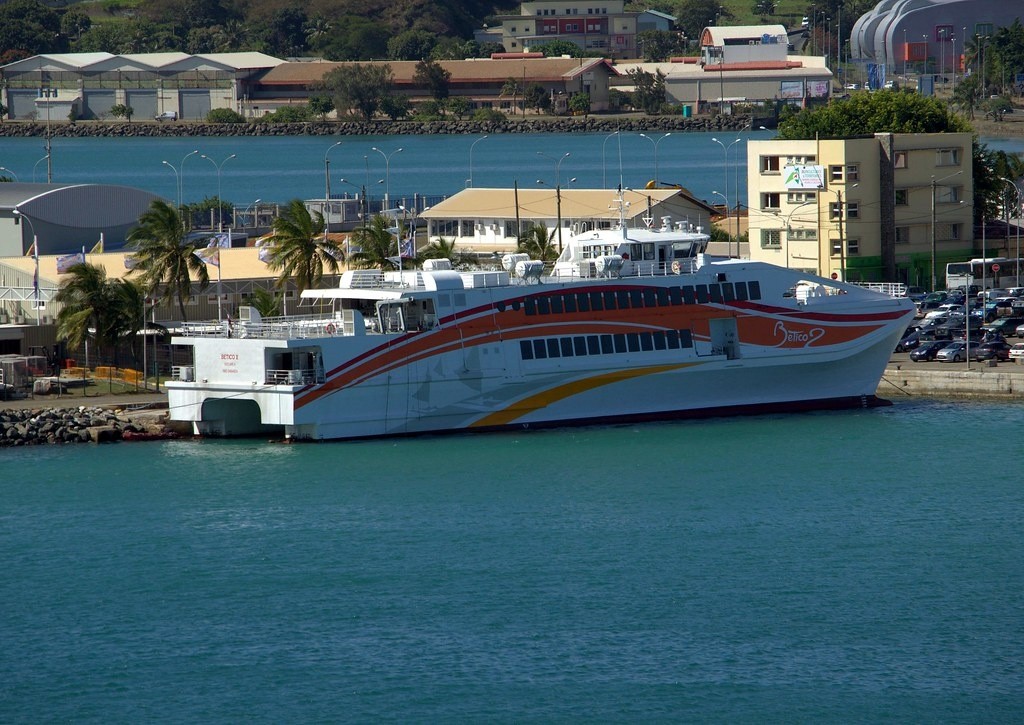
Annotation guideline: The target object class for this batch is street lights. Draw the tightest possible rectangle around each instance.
[811,3,850,93]
[12,210,39,324]
[398,205,429,251]
[711,123,749,260]
[603,131,620,189]
[536,152,577,255]
[640,133,671,188]
[931,170,964,291]
[773,202,812,269]
[469,136,489,188]
[162,150,260,230]
[341,147,402,226]
[324,141,343,234]
[1000,177,1020,287]
[817,183,859,283]
[33,155,50,184]
[904,26,985,97]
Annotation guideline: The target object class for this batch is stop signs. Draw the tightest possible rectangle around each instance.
[992,263,999,271]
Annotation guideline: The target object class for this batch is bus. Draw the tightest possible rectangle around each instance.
[946,258,1024,290]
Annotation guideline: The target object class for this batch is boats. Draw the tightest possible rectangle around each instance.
[162,187,917,442]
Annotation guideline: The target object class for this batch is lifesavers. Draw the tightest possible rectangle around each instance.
[327,324,335,335]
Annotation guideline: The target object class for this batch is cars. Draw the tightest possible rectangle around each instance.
[846,81,899,90]
[895,286,1024,351]
[909,340,1024,363]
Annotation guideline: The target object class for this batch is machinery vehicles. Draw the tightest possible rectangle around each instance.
[24,346,56,376]
